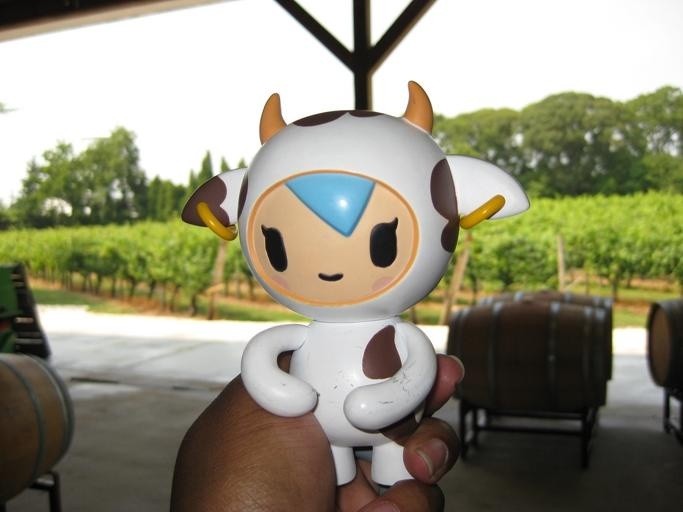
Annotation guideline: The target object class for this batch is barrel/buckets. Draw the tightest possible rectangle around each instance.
[470,290,613,381]
[446,299,606,412]
[0,353,74,505]
[645,300,683,389]
